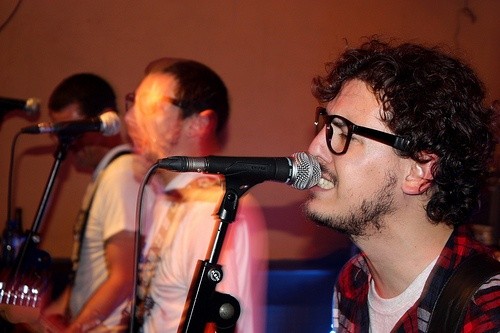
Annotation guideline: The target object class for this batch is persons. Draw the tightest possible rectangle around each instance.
[303,38,500,333]
[40,72,164,333]
[87,57,268,333]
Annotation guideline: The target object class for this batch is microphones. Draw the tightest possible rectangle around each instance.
[0,97,41,120]
[23,110,121,137]
[158,150,321,190]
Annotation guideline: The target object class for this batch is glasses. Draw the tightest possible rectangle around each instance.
[313,107,396,156]
[125,93,180,117]
